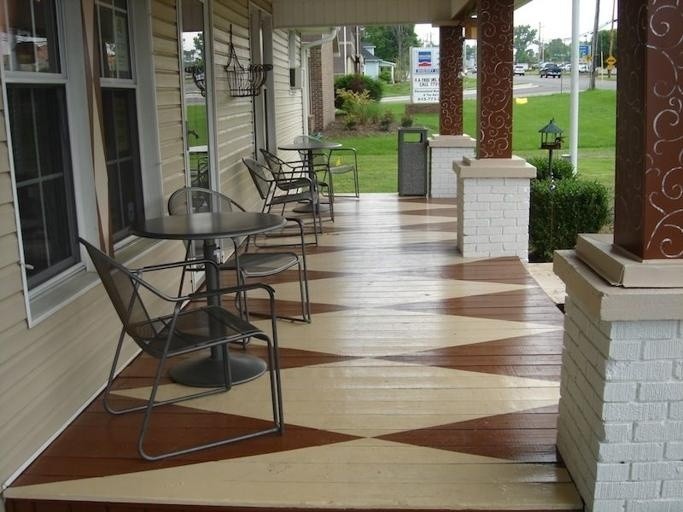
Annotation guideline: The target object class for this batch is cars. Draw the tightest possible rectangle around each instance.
[513,65,525,75]
[539,64,562,78]
[558,62,590,73]
[465,66,478,75]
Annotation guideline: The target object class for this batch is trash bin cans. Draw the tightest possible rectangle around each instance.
[398,126,428,196]
